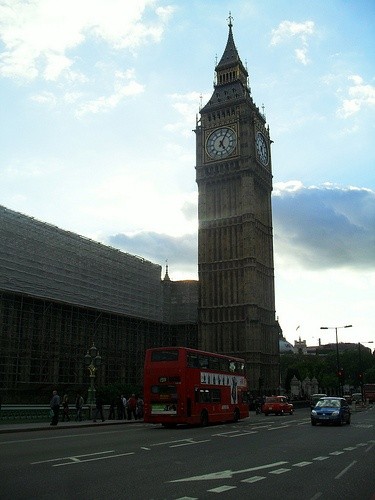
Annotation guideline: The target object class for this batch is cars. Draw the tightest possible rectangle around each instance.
[342,392,362,405]
[261,395,295,416]
[310,396,352,426]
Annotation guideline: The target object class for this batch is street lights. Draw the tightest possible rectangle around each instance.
[344,341,374,394]
[82,340,103,420]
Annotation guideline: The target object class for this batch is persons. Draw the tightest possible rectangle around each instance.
[108,392,143,420]
[50,390,60,426]
[255,394,265,415]
[75,392,84,421]
[59,394,70,422]
[93,392,105,422]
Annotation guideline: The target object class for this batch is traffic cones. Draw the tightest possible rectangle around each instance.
[320,324,353,395]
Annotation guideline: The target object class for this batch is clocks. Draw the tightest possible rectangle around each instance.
[204,126,238,158]
[256,129,270,168]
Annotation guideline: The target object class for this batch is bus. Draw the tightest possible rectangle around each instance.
[362,384,375,404]
[143,346,249,428]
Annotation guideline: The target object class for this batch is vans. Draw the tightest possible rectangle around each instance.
[309,393,327,412]
